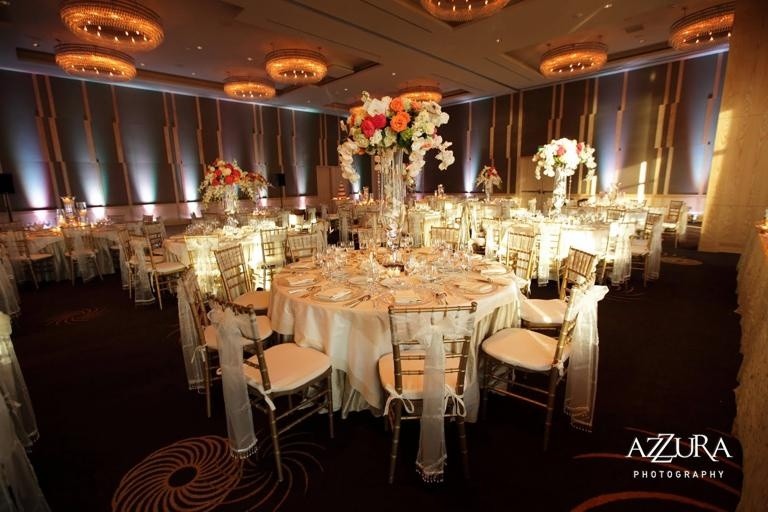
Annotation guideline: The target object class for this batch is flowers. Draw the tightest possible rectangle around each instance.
[337,91,455,189]
[198,158,268,210]
[531,137,597,183]
[476,166,502,189]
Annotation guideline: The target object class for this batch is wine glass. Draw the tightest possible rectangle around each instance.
[24,201,113,232]
[273,240,513,303]
[181,206,289,236]
[362,184,645,227]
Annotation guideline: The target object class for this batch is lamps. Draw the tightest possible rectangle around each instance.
[421,0,509,21]
[60,1,165,51]
[669,1,735,50]
[223,77,275,99]
[538,42,608,80]
[54,44,136,81]
[265,49,328,85]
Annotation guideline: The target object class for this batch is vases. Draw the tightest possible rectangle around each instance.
[382,148,403,250]
[484,182,494,202]
[225,193,237,228]
[552,168,566,220]
